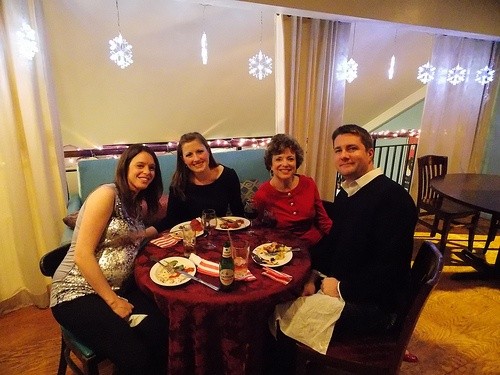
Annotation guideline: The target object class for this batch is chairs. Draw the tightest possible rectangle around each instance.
[38,242,113,375]
[415,155,473,253]
[293,241,443,375]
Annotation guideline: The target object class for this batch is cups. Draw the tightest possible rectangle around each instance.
[232,240,250,284]
[183,225,197,257]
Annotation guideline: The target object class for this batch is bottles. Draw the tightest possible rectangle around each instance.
[218,241,236,292]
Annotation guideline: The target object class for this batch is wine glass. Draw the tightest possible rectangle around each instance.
[201,209,218,249]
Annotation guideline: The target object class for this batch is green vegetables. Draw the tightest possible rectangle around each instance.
[166,261,184,271]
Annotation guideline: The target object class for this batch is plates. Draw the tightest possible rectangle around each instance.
[209,216,251,230]
[251,243,293,268]
[170,220,209,239]
[149,257,197,286]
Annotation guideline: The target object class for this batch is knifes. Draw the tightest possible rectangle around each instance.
[175,268,220,291]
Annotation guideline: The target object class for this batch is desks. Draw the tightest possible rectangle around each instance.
[431,173,500,282]
[133,219,311,375]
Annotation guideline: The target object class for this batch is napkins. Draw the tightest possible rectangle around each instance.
[150,233,180,249]
[196,259,221,277]
[261,267,293,284]
[268,289,346,355]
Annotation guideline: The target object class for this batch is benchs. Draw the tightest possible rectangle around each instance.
[60,147,272,245]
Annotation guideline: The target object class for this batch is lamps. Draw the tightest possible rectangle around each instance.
[343,20,358,84]
[200,5,208,66]
[388,28,398,80]
[418,35,495,86]
[248,8,273,80]
[109,0,134,70]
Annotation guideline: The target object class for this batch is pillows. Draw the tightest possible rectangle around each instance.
[240,178,263,207]
[62,211,77,229]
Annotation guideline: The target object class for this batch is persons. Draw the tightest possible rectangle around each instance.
[260,124,418,375]
[166,132,243,229]
[251,133,332,247]
[49,143,164,375]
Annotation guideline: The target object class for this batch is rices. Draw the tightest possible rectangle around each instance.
[158,269,185,284]
